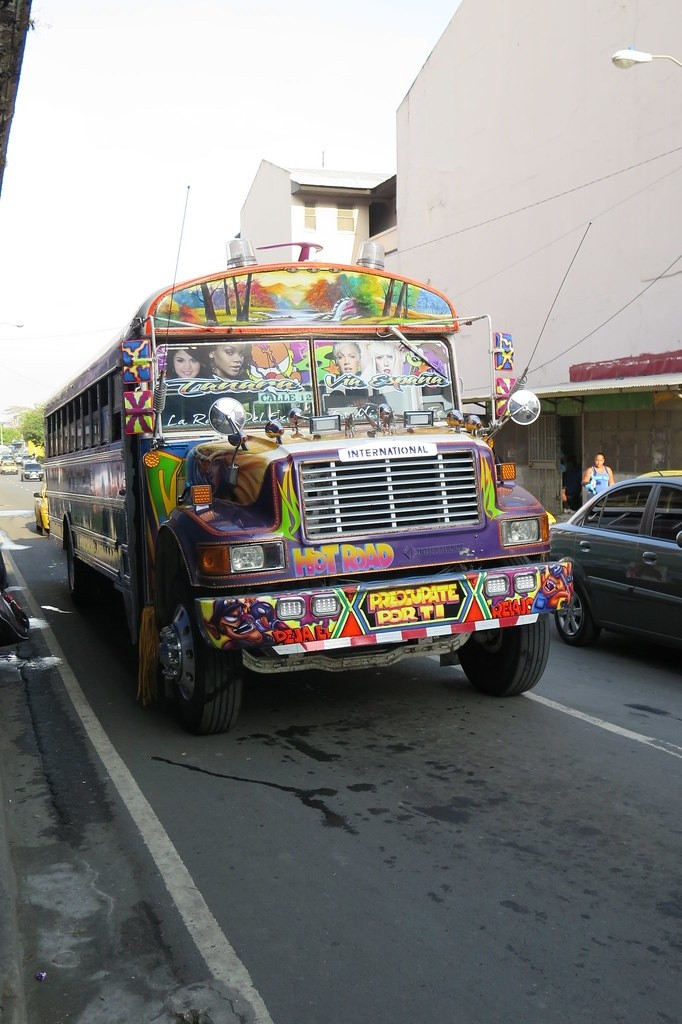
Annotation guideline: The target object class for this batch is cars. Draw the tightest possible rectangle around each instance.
[634,469,682,478]
[21,463,44,481]
[15,453,28,463]
[0,455,14,461]
[33,481,59,537]
[542,510,556,527]
[546,478,682,645]
[20,455,36,466]
[0,461,18,475]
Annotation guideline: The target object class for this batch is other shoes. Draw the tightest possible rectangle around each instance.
[564,508,573,513]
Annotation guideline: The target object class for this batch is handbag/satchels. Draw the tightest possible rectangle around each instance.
[0,589,29,647]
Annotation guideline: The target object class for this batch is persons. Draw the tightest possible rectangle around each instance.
[208,344,248,379]
[167,350,214,380]
[366,342,401,375]
[582,452,615,501]
[562,451,573,512]
[332,343,362,377]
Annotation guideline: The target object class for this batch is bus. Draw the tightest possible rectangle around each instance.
[42,267,570,733]
